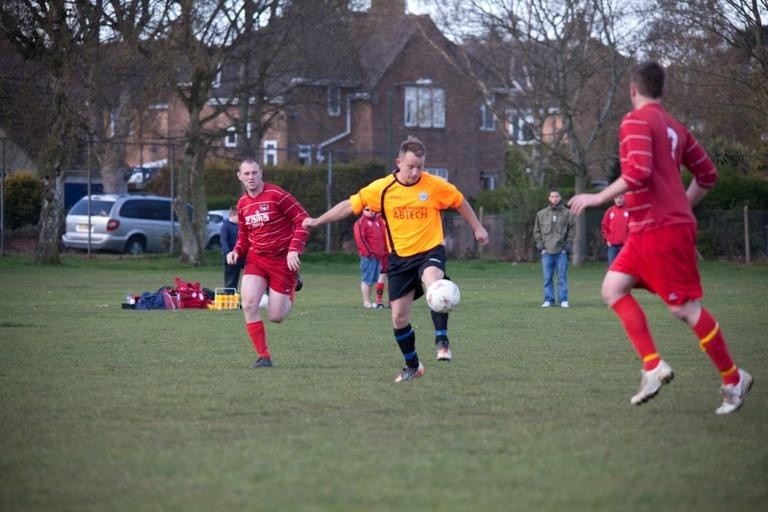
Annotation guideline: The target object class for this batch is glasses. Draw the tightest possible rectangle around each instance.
[362,208,368,211]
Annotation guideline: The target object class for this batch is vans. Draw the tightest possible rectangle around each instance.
[61,193,222,253]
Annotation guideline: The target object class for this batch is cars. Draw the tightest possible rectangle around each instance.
[208,209,231,225]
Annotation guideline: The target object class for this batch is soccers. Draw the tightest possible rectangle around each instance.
[424,279,461,314]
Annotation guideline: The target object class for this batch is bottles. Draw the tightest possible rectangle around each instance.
[217,292,223,310]
[227,291,233,309]
[223,292,228,310]
[233,290,240,308]
[214,293,219,306]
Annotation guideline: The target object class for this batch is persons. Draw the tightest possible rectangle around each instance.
[567,61,753,416]
[601,194,629,269]
[225,159,311,369]
[531,190,576,309]
[302,139,490,382]
[352,205,384,310]
[220,206,244,296]
[373,212,396,309]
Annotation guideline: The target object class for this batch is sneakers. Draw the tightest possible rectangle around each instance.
[715,369,752,415]
[295,277,303,292]
[629,360,674,406]
[436,341,450,361]
[541,302,550,308]
[251,357,272,367]
[395,362,424,384]
[376,301,383,309]
[560,301,568,307]
